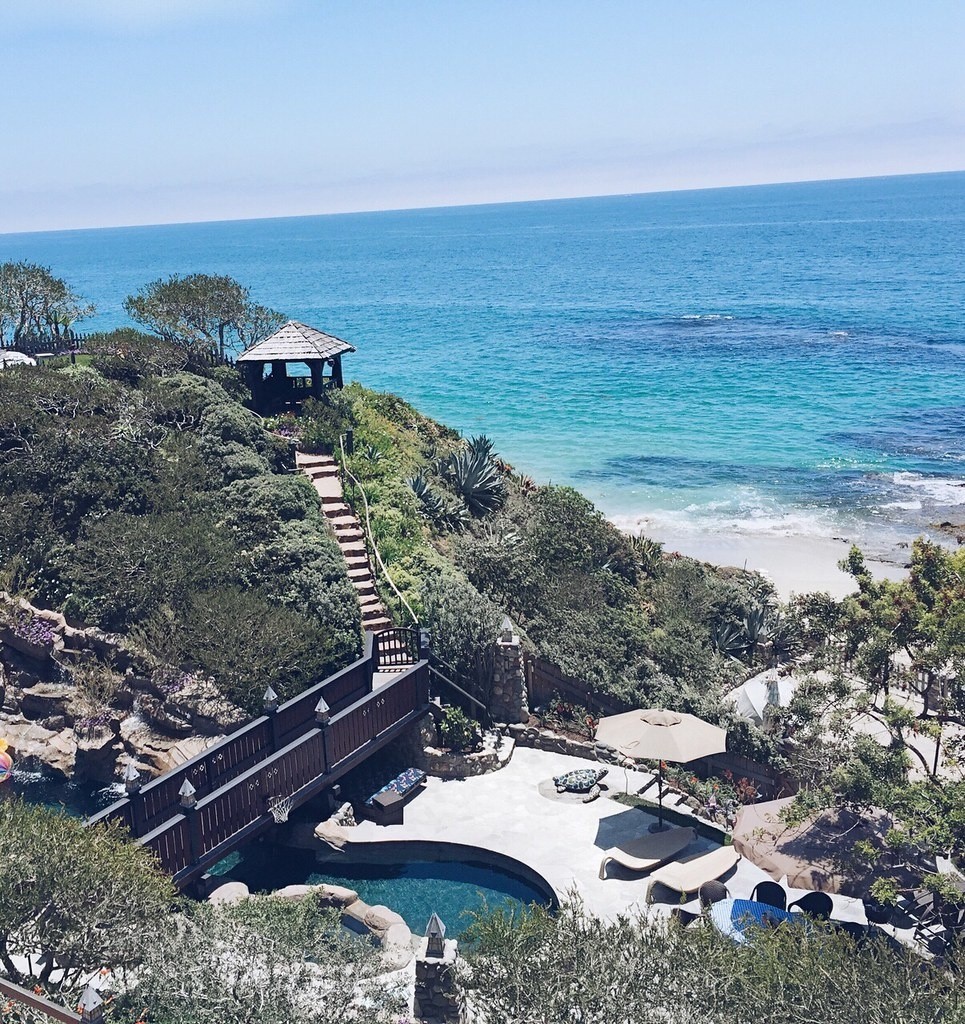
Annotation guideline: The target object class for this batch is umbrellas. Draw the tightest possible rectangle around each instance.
[595,709,727,826]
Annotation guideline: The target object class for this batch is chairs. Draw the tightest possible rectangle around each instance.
[646,843,741,906]
[672,908,700,926]
[697,880,731,914]
[598,826,698,879]
[787,891,833,919]
[749,881,787,911]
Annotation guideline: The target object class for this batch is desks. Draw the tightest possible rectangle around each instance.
[711,899,805,956]
